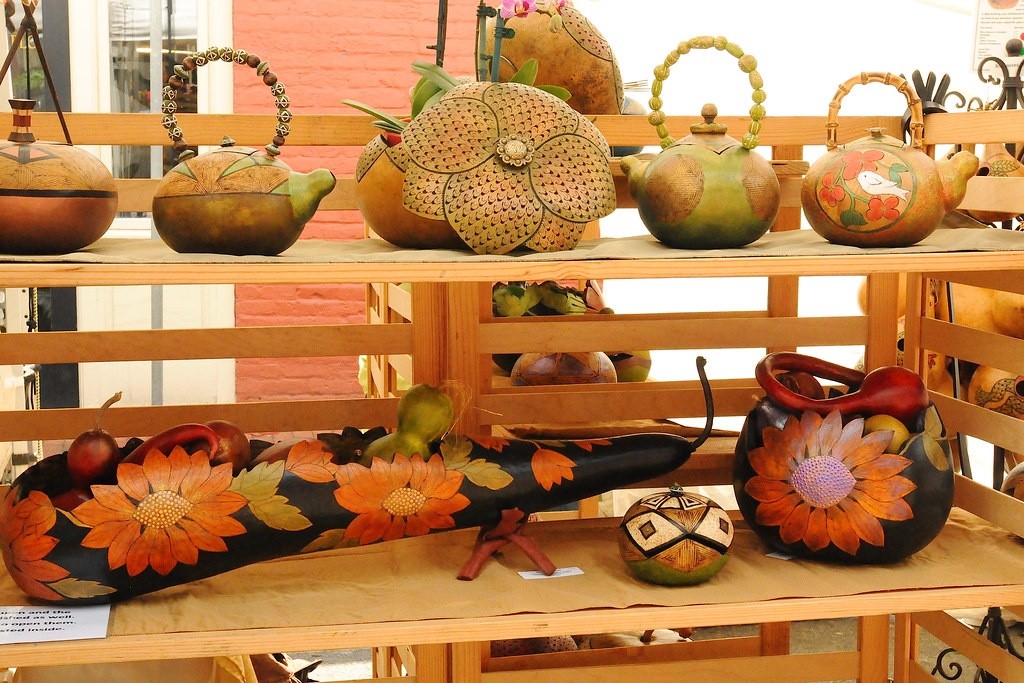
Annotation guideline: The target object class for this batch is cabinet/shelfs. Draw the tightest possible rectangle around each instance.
[0,109,1024,683]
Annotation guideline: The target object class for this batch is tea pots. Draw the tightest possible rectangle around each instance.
[151,45,335,256]
[800,75,979,249]
[616,37,781,251]
